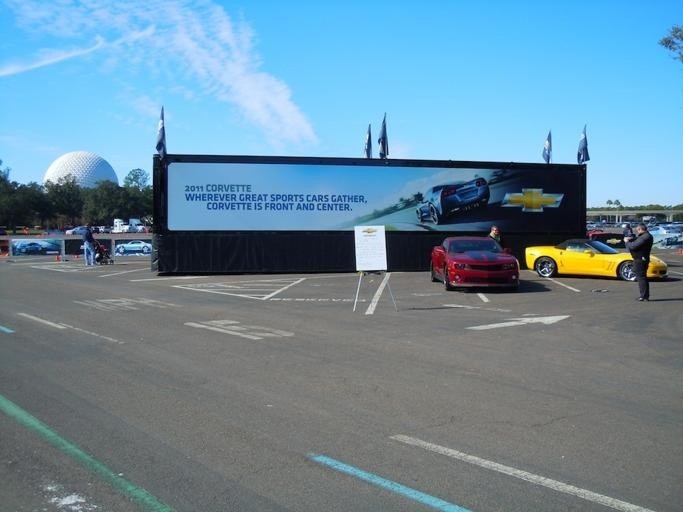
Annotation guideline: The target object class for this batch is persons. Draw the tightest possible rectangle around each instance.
[624,224,633,250]
[487,225,505,248]
[83,222,97,267]
[99,243,108,252]
[623,223,654,301]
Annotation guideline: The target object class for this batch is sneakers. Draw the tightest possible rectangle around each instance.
[85,264,97,266]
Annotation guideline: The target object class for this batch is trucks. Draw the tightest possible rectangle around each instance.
[65,218,146,235]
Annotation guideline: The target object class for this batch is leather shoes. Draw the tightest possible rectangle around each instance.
[635,296,649,301]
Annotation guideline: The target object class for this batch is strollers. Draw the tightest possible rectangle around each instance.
[94,240,114,265]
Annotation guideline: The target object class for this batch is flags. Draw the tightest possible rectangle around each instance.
[378,114,389,158]
[155,105,166,161]
[362,122,371,159]
[576,124,590,163]
[541,130,553,162]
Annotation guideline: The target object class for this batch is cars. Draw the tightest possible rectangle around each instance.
[431,236,520,291]
[116,241,153,254]
[586,216,683,249]
[415,176,490,224]
[525,238,669,282]
[17,242,47,254]
